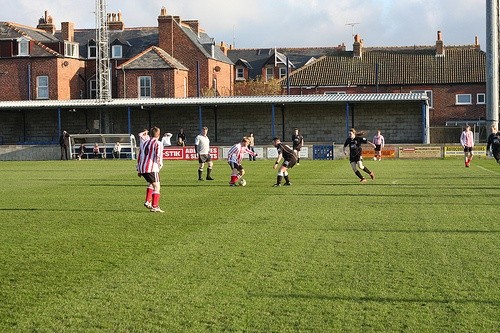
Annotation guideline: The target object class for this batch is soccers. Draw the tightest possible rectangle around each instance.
[238,178,246,187]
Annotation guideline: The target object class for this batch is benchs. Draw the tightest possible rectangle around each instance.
[71,147,135,160]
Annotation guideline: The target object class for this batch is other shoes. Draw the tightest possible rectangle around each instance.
[370,172,375,180]
[283,182,290,186]
[229,182,238,186]
[274,184,281,187]
[361,179,366,183]
[466,159,470,167]
[297,163,299,165]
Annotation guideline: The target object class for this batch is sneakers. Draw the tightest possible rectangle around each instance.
[206,176,213,180]
[199,178,204,181]
[151,207,165,212]
[144,201,153,209]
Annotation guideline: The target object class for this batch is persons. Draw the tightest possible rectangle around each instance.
[112,141,122,159]
[93,144,100,158]
[460,125,474,167]
[59,131,71,160]
[272,128,303,187]
[227,132,258,186]
[137,126,173,213]
[372,130,385,161]
[486,124,500,166]
[194,127,214,180]
[77,143,86,161]
[177,128,186,147]
[342,128,376,182]
